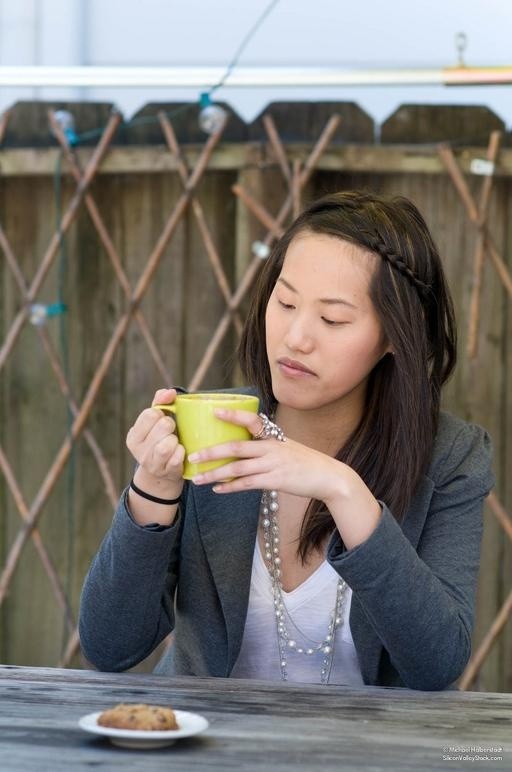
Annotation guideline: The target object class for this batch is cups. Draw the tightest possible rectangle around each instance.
[148,391,261,489]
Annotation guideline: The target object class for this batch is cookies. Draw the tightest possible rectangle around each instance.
[97,704,178,731]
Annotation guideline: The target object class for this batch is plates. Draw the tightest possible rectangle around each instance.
[79,707,210,748]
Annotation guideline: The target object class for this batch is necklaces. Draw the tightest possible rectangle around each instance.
[261,488,348,685]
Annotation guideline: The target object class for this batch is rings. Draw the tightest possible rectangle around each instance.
[254,412,287,443]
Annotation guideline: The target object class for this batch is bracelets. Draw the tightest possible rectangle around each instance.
[130,478,184,505]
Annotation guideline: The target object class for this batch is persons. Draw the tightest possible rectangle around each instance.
[78,192,493,694]
